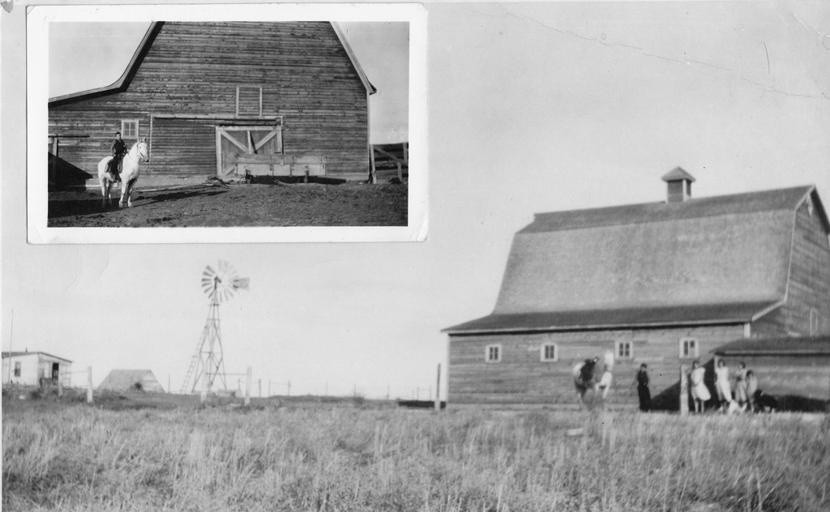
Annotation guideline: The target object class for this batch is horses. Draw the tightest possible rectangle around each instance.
[96,137,151,209]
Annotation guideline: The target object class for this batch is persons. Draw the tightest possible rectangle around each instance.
[636,363,653,413]
[689,357,712,416]
[110,132,129,184]
[733,361,748,413]
[715,358,734,413]
[745,369,758,412]
